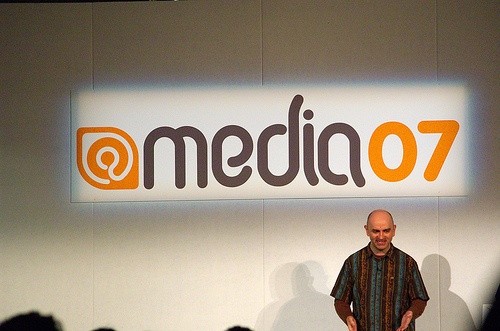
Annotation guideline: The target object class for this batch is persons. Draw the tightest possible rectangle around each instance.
[330,209,430,331]
[0,310,63,331]
[226,325,253,331]
[89,327,116,331]
